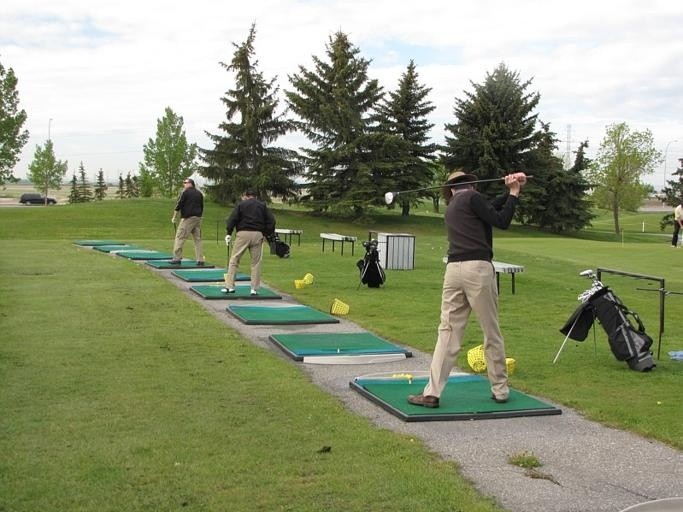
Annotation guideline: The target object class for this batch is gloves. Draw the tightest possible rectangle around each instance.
[172,218,178,224]
[225,235,231,246]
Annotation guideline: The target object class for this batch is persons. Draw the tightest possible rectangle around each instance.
[218,188,280,297]
[669,203,682,248]
[406,170,528,408]
[167,178,204,268]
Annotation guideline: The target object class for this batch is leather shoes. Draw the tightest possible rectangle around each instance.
[168,261,181,265]
[196,261,204,266]
[491,394,506,403]
[408,395,439,408]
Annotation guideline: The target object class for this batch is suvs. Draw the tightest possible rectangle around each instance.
[19,193,57,206]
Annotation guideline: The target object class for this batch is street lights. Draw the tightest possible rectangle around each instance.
[47,118,54,140]
[663,139,680,190]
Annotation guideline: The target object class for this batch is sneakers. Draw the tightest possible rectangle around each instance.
[221,288,235,293]
[250,289,257,296]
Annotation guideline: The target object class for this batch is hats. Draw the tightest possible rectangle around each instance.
[445,171,478,189]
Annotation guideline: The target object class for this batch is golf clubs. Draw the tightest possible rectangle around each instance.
[356,239,385,292]
[385,175,534,205]
[553,268,603,363]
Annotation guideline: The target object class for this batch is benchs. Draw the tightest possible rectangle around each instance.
[274,229,303,247]
[320,233,357,257]
[443,256,524,295]
